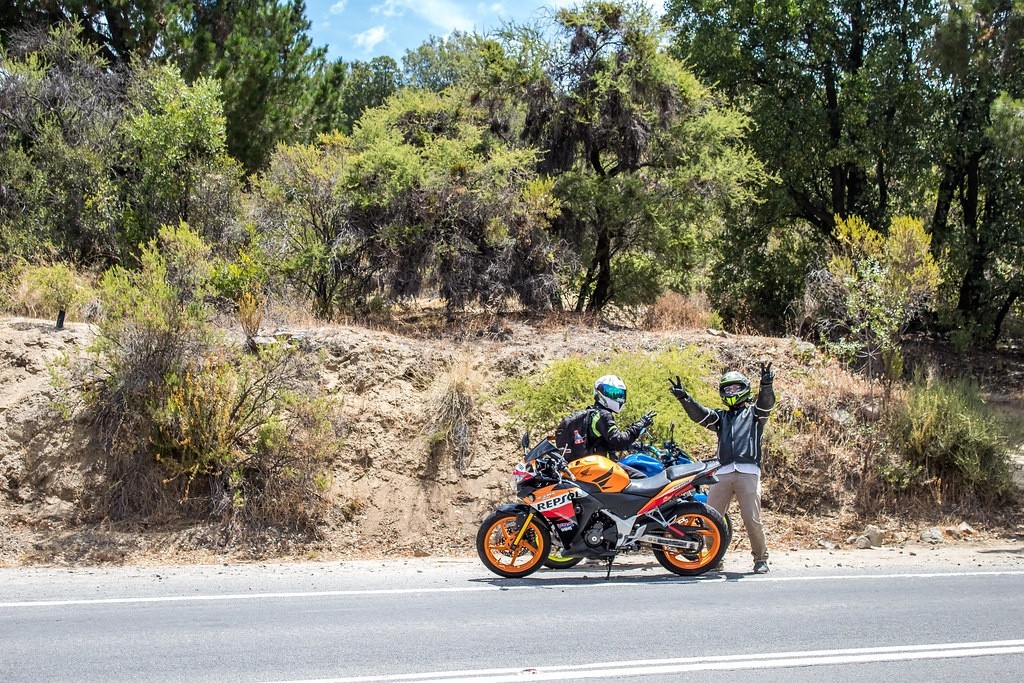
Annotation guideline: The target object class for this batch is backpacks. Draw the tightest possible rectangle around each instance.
[555,410,590,461]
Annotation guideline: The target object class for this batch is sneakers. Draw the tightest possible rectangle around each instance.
[753,561,768,574]
[710,563,724,571]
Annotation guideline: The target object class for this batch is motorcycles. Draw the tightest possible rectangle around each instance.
[475,433,729,578]
[528,430,734,569]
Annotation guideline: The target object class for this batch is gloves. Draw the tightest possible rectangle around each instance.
[667,375,687,399]
[639,410,657,428]
[761,362,776,382]
[631,441,645,452]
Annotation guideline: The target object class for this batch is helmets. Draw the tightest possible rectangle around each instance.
[718,370,751,408]
[593,375,627,415]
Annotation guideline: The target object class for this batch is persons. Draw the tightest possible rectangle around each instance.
[555,374,658,564]
[668,361,776,575]
